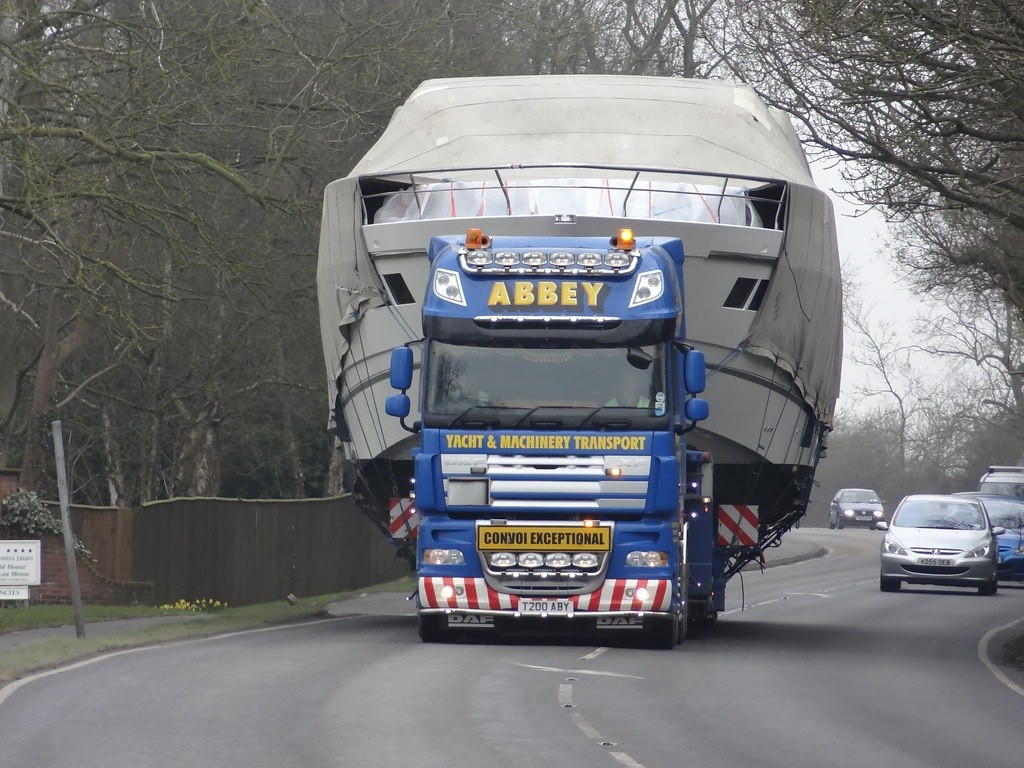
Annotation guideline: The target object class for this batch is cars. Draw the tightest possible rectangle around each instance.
[952,492,1024,580]
[875,493,1005,595]
[829,489,886,529]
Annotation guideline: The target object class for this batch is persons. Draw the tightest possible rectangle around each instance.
[605,380,650,407]
[430,362,488,415]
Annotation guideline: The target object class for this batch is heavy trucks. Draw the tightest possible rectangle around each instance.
[385,229,760,649]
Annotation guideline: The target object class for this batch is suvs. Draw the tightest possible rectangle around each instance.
[977,466,1024,498]
